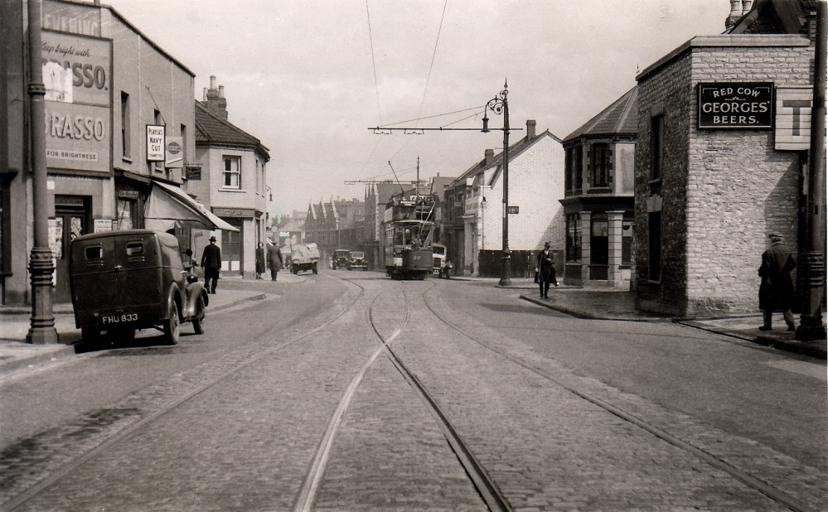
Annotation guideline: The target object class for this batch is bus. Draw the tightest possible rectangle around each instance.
[377,219,435,280]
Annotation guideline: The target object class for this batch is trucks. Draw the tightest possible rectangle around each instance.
[431,243,452,279]
[291,243,320,275]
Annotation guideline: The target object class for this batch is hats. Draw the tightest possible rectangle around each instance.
[545,242,550,248]
[769,231,785,237]
[210,236,216,242]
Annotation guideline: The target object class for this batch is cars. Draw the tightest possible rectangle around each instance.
[331,248,369,271]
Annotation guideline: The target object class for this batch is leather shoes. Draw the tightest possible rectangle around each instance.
[759,327,771,330]
[541,294,548,299]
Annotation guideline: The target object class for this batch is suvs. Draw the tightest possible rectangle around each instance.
[67,229,209,345]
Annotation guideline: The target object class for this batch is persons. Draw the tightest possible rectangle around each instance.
[534,242,558,298]
[256,242,265,280]
[201,236,221,294]
[758,232,796,331]
[267,241,283,281]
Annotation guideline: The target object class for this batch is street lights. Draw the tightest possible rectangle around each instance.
[481,77,512,286]
